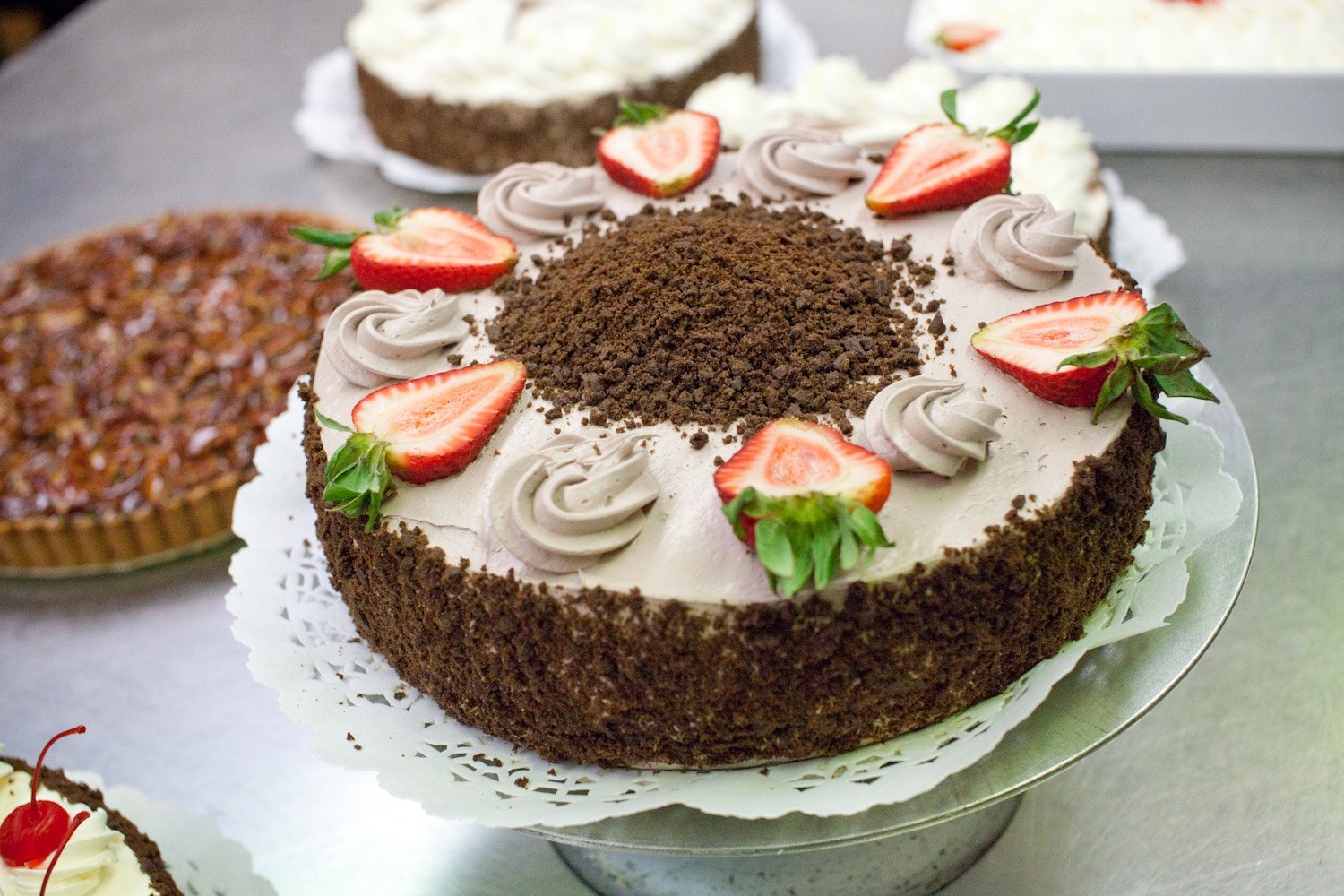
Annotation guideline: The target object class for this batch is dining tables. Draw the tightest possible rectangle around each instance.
[0,0,1344,896]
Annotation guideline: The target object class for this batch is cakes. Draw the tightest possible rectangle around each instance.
[683,55,1115,258]
[345,0,761,175]
[0,209,382,568]
[295,72,1222,771]
[0,725,182,896]
[905,0,1344,149]
